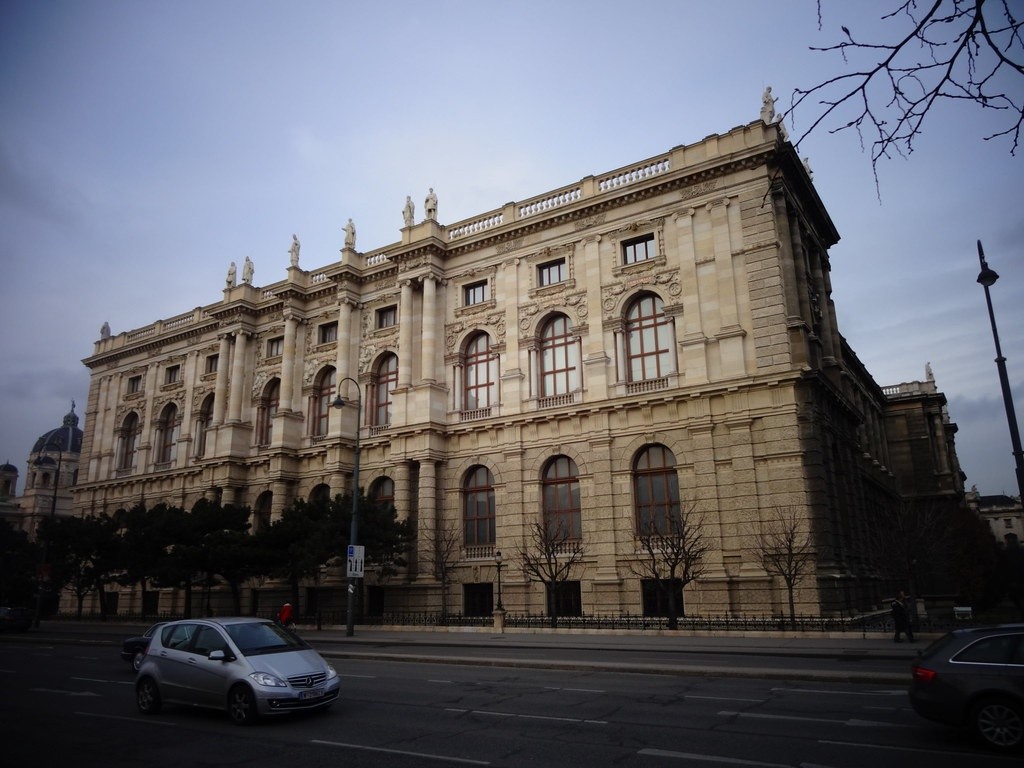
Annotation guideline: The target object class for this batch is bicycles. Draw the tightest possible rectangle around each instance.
[276,612,296,632]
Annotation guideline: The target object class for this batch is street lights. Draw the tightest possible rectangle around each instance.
[332,376,361,637]
[491,550,508,635]
[976,239,1023,504]
[29,442,62,628]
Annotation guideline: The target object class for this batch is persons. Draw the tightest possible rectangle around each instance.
[277,598,292,626]
[891,588,917,644]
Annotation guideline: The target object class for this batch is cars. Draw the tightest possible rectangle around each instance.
[121,621,194,675]
[0,588,36,634]
[136,617,342,727]
[908,623,1024,756]
